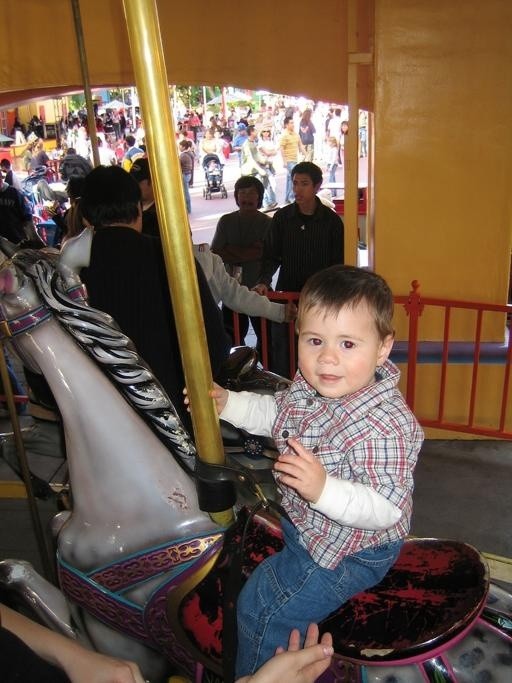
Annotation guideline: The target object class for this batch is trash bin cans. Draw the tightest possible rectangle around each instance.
[316,182,369,249]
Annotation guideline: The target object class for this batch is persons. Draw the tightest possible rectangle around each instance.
[78,159,232,446]
[250,161,344,381]
[0,602,336,682]
[0,101,147,249]
[178,260,426,682]
[175,95,369,211]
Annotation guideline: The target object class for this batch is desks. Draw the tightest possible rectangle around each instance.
[46,160,64,182]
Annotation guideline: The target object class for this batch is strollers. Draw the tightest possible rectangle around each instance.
[202,153,227,199]
